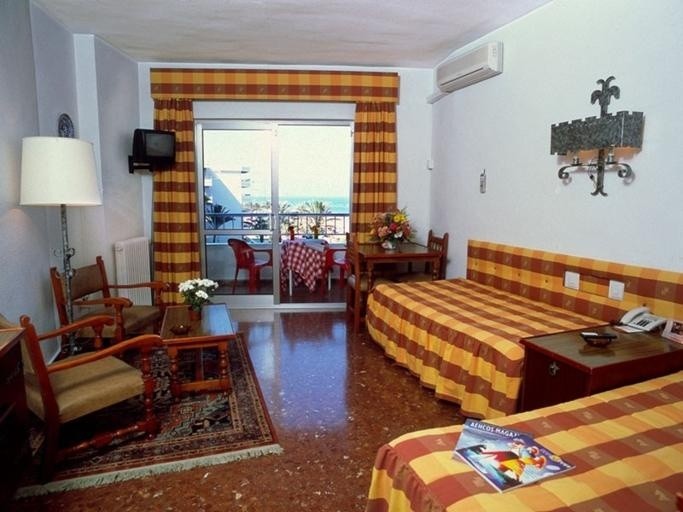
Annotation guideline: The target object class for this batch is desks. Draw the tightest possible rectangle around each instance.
[279,240,331,297]
[518,322,683,412]
[0,328,27,511]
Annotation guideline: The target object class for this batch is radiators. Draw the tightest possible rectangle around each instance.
[114,237,151,306]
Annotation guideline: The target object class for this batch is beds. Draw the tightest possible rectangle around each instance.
[366,279,610,420]
[392,373,682,512]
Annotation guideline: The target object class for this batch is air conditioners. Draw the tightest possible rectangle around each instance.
[436,41,503,92]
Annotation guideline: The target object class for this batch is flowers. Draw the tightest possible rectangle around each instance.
[369,208,416,245]
[177,278,219,311]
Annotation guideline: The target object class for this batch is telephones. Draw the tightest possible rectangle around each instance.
[480,169,486,193]
[620,306,667,332]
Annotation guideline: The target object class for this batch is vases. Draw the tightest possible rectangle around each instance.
[187,305,202,320]
[381,240,395,250]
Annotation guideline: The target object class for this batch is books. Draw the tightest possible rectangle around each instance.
[452,417,534,469]
[454,432,576,496]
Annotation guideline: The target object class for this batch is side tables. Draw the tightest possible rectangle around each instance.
[358,243,441,292]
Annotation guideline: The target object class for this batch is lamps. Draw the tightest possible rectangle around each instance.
[18,136,103,358]
[550,76,644,196]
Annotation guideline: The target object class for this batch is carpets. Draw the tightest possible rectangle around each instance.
[16,331,284,496]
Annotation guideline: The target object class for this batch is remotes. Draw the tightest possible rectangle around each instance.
[582,332,617,338]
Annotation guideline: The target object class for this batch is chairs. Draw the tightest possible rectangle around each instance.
[345,233,365,334]
[227,238,272,294]
[20,314,165,470]
[319,247,347,297]
[426,230,448,279]
[50,256,171,360]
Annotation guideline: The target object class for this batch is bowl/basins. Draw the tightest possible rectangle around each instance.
[580,330,617,348]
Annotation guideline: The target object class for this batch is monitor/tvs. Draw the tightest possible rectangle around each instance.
[133,129,176,164]
